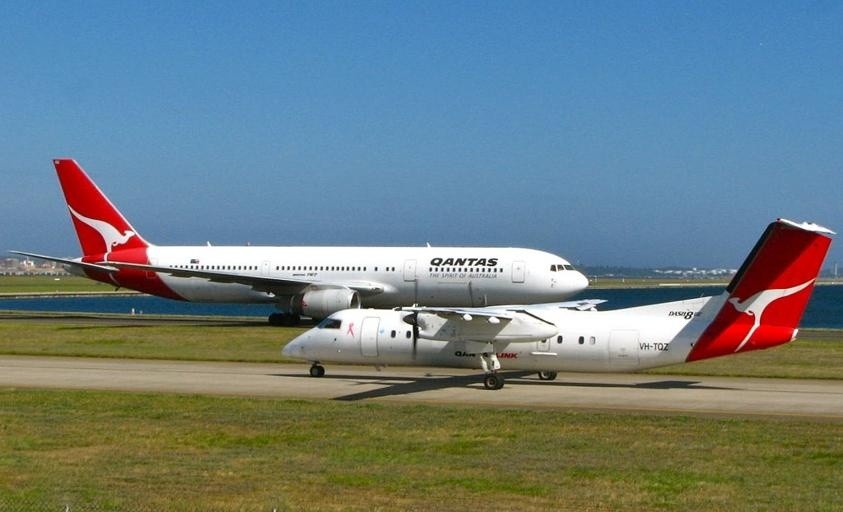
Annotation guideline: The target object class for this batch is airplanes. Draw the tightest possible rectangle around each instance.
[8,157,588,328]
[278,213,837,392]
